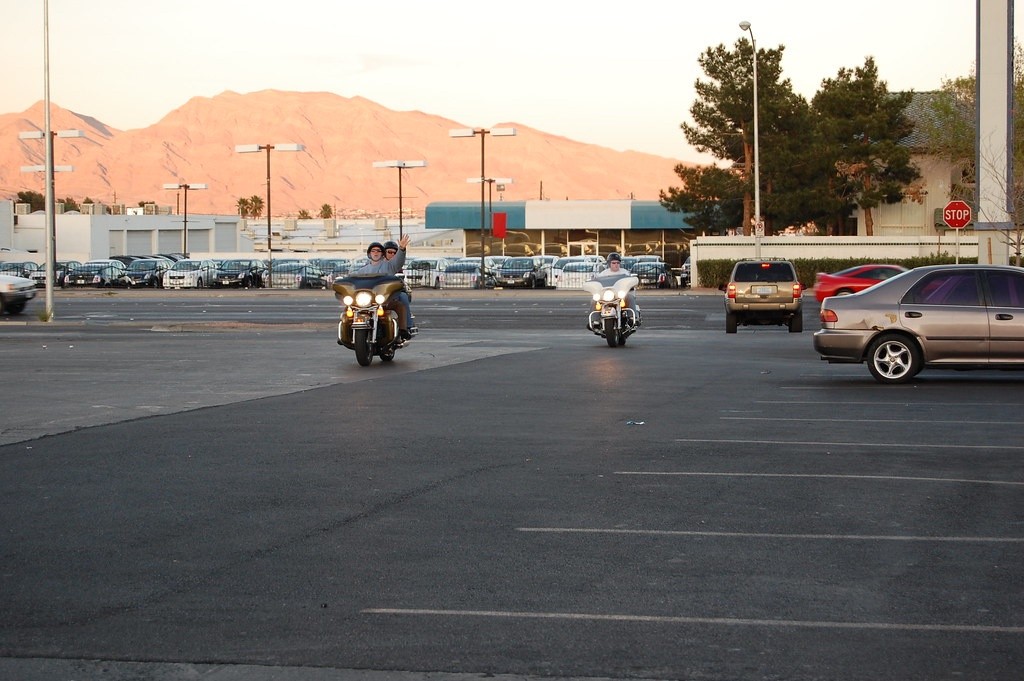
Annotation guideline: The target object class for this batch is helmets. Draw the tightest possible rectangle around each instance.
[367,242,384,259]
[607,253,621,263]
[383,241,399,251]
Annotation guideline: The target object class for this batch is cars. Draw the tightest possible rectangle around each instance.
[813,265,918,302]
[0,262,38,278]
[24,229,691,290]
[1,270,37,317]
[813,263,1024,384]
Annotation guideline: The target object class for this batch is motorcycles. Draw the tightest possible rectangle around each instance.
[581,270,643,347]
[330,268,419,369]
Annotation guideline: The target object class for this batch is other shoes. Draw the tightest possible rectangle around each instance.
[628,318,633,326]
[337,339,343,346]
[587,322,601,330]
[399,330,411,340]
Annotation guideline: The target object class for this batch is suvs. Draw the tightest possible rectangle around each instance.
[718,260,808,333]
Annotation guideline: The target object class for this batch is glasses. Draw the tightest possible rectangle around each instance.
[370,249,383,252]
[610,262,620,265]
[386,251,397,255]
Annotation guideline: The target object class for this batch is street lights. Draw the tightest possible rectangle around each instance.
[448,127,517,288]
[737,20,765,263]
[465,177,514,254]
[17,131,83,288]
[234,143,303,291]
[372,160,429,272]
[585,230,599,274]
[161,182,209,259]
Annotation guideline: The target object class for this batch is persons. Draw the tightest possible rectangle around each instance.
[383,241,413,334]
[337,233,412,346]
[588,252,639,328]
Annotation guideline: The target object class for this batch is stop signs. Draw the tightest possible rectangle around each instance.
[942,199,974,230]
